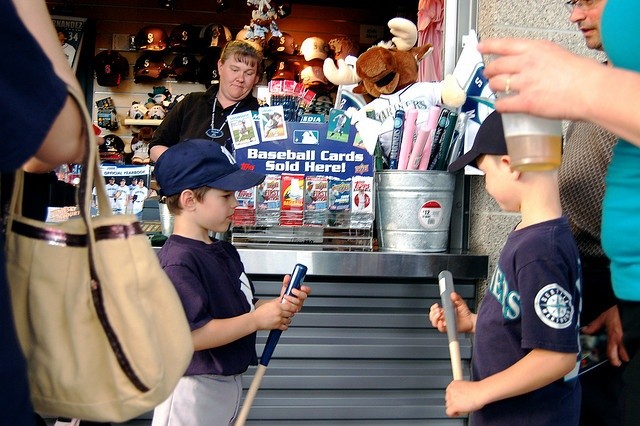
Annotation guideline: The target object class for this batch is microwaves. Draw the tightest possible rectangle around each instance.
[435,110,458,171]
[400,110,417,170]
[430,109,449,170]
[438,271,469,419]
[387,109,404,169]
[236,265,308,425]
[408,126,430,171]
[420,105,442,170]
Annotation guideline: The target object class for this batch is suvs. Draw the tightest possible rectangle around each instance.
[377,169,457,253]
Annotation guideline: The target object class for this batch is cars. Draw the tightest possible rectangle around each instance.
[488,56,562,172]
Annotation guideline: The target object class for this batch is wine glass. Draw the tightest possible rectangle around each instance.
[505,74,511,94]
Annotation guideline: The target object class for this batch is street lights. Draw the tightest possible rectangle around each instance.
[4,83,193,423]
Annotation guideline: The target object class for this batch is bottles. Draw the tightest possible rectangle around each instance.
[300,37,327,61]
[170,56,199,83]
[155,140,266,197]
[200,24,231,48]
[96,50,129,86]
[201,52,221,89]
[267,32,298,55]
[266,60,296,81]
[300,66,328,84]
[169,25,199,49]
[134,53,169,78]
[138,27,168,50]
[98,134,124,160]
[448,109,562,173]
[235,29,262,51]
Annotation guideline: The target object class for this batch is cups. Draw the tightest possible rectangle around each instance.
[566,0,597,11]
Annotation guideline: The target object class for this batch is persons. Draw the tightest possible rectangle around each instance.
[358,189,366,210]
[57,30,76,67]
[236,120,253,145]
[152,139,311,426]
[428,108,584,426]
[330,112,346,137]
[476,0,640,426]
[557,0,633,426]
[329,184,339,209]
[92,186,99,217]
[148,40,261,163]
[128,178,138,214]
[305,182,314,210]
[113,179,130,215]
[105,177,119,215]
[257,182,270,205]
[0,0,104,426]
[130,179,148,214]
